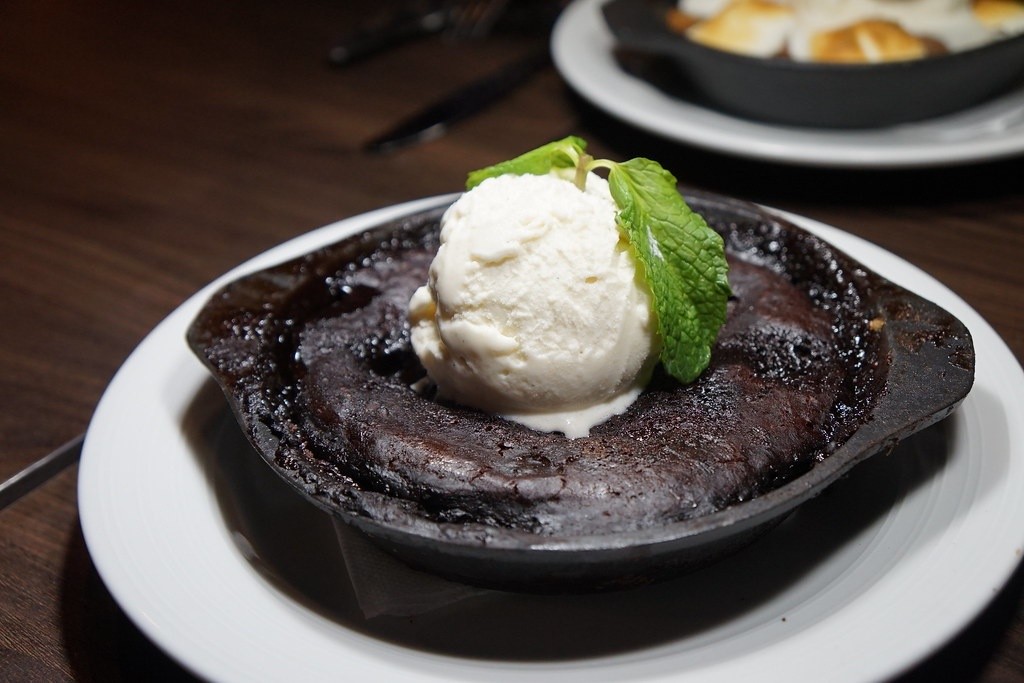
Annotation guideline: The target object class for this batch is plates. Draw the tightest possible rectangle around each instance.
[78,190,1022,683]
[550,0,1024,166]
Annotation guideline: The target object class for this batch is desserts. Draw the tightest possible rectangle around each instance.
[277,134,858,542]
